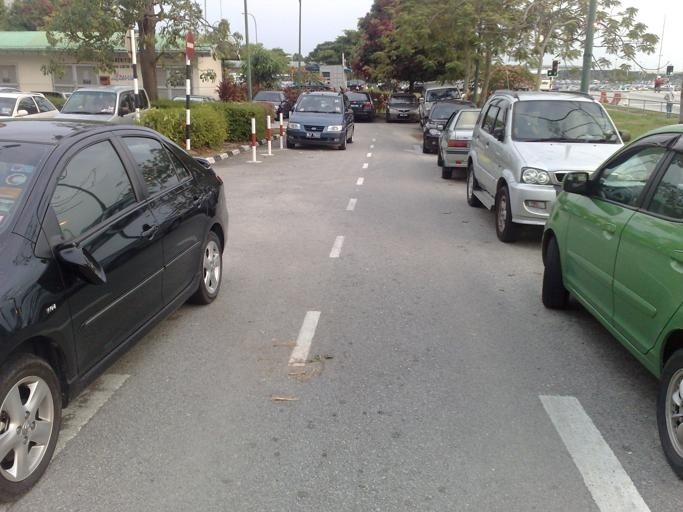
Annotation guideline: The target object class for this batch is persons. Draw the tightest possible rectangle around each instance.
[664,86,675,119]
[654,75,663,93]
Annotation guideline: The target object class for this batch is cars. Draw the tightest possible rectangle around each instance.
[539,123,683,483]
[287,92,354,150]
[0,117,228,504]
[344,92,375,122]
[59,86,151,119]
[0,92,59,117]
[423,100,481,179]
[252,90,290,121]
[467,91,625,242]
[386,93,420,122]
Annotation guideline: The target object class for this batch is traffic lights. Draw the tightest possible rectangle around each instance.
[547,60,558,77]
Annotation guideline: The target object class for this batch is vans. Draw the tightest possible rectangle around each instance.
[419,85,463,126]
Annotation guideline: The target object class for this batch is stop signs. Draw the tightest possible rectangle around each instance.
[185,32,196,60]
